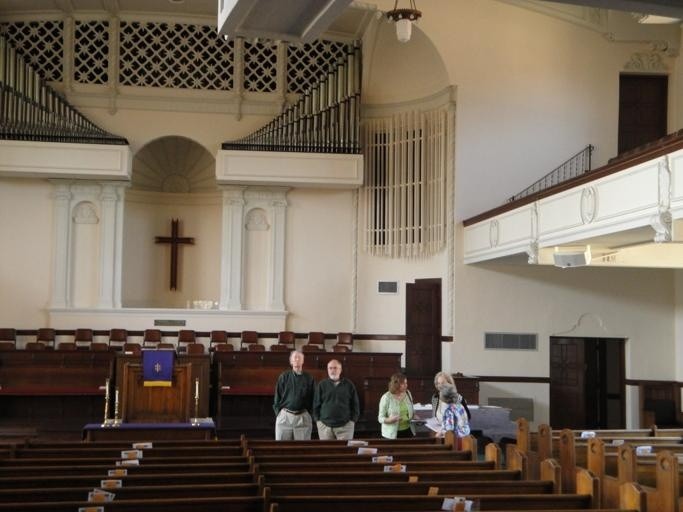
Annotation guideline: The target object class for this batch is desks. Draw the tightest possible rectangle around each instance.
[361,376,480,422]
[412,404,515,444]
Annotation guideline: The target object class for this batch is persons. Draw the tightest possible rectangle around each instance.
[431,371,471,437]
[272,350,316,441]
[377,373,424,440]
[311,358,361,440]
[433,384,470,437]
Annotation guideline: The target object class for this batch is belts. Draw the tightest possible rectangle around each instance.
[284,409,304,416]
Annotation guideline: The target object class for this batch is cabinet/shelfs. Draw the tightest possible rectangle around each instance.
[1,351,115,420]
[217,352,402,431]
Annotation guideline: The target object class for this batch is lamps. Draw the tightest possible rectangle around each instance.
[385,0,421,44]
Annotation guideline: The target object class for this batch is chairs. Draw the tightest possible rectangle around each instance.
[209,331,354,371]
[1,328,205,353]
